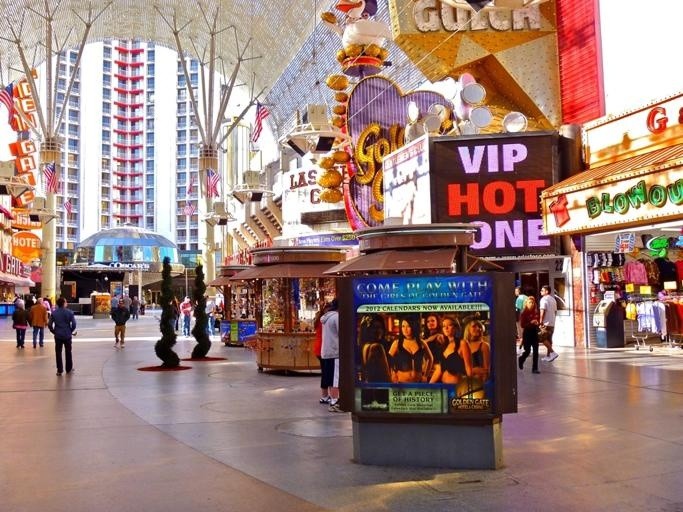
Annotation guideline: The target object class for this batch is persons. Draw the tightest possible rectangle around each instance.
[47,298,76,376]
[517,295,540,375]
[514,286,528,339]
[537,284,558,362]
[321,298,339,410]
[358,311,491,384]
[12,292,224,349]
[312,303,329,404]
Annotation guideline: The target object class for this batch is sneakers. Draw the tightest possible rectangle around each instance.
[113,343,118,347]
[519,356,526,370]
[532,369,540,374]
[319,396,345,413]
[121,345,125,348]
[540,351,559,362]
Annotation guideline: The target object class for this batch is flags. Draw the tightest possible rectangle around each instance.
[186,175,195,195]
[43,161,57,193]
[0,82,14,123]
[181,201,196,216]
[250,101,270,142]
[63,197,72,216]
[207,169,220,198]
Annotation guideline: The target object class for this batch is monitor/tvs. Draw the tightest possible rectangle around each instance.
[336,272,517,425]
